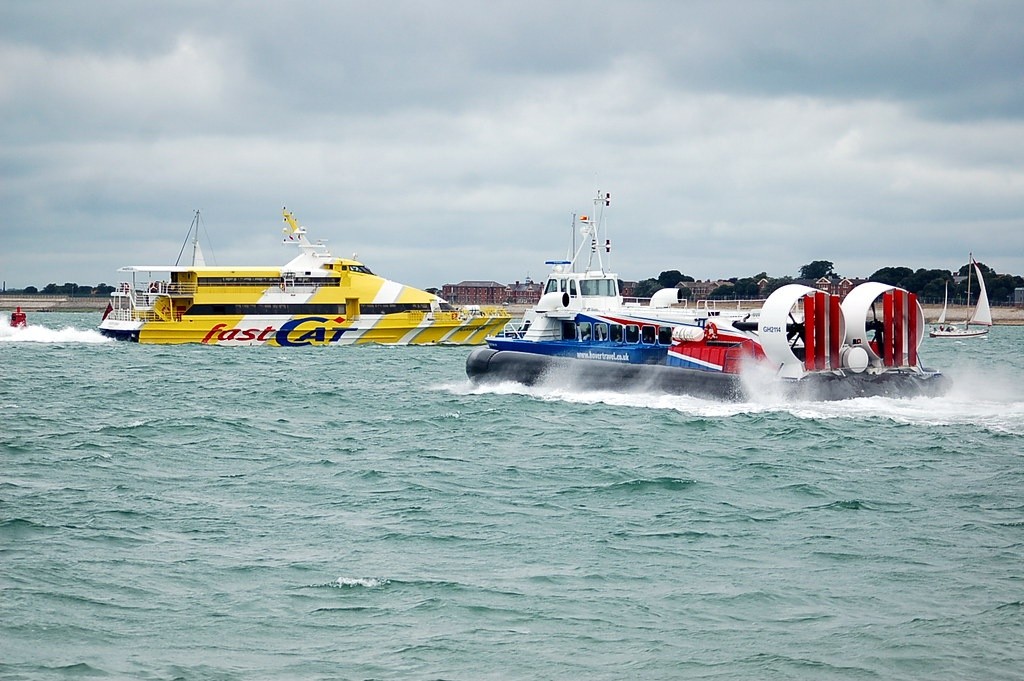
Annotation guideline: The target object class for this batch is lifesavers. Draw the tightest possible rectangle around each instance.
[451,312,458,320]
[162,306,170,315]
[703,321,718,340]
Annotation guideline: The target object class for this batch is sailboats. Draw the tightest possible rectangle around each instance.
[930,252,994,338]
[929,280,951,325]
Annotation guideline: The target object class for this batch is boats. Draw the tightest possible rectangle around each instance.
[97,204,511,350]
[462,187,959,404]
